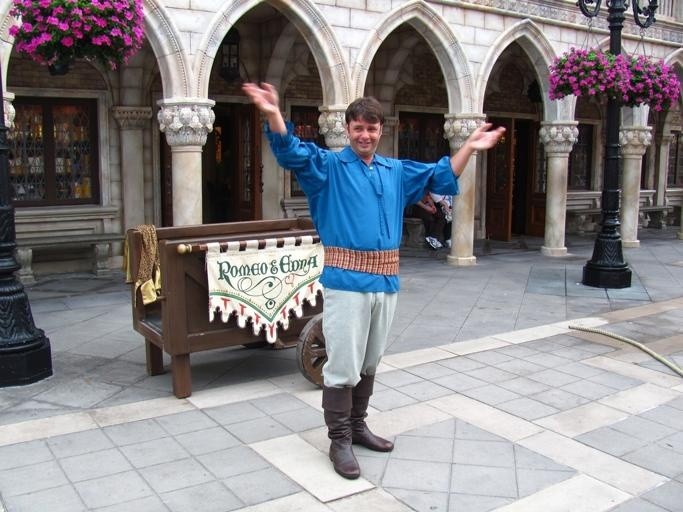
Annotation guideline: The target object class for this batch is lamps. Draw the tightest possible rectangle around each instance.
[219,27,241,80]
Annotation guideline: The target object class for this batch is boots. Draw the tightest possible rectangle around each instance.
[321,384,361,479]
[352,374,394,451]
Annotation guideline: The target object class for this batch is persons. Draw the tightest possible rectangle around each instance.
[414,192,453,250]
[241,80,506,480]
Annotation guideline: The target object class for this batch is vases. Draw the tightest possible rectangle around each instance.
[47,53,70,76]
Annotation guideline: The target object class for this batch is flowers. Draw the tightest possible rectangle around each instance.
[8,0,146,72]
[545,49,682,114]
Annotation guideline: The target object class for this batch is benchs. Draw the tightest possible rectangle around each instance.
[567,205,672,238]
